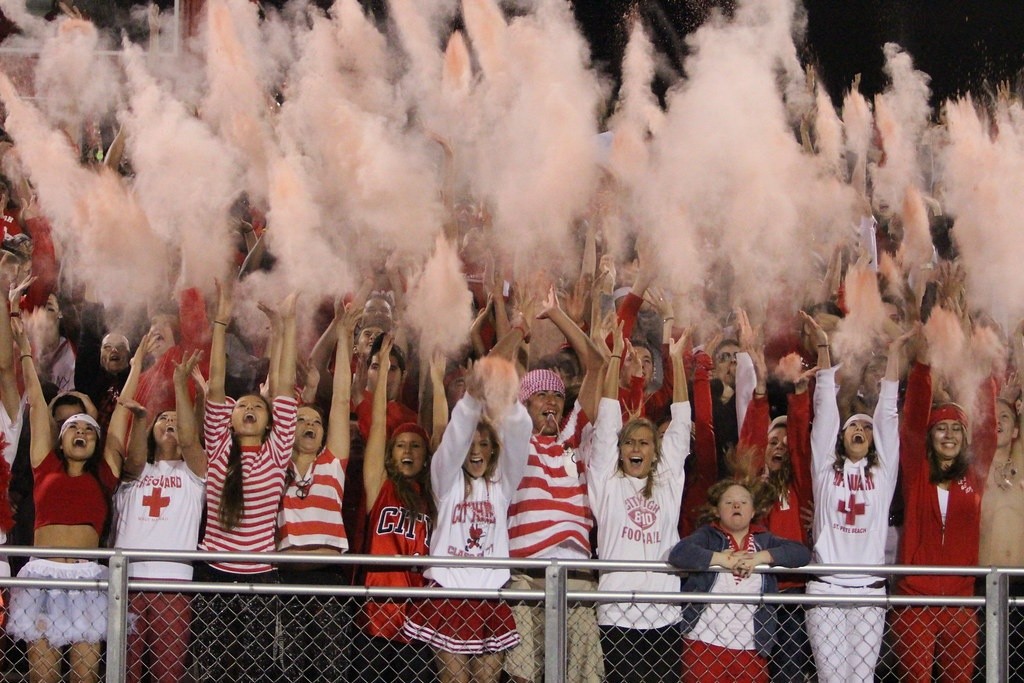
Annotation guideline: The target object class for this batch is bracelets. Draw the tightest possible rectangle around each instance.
[611,355,621,358]
[818,344,829,347]
[19,355,32,361]
[513,326,525,339]
[663,317,674,322]
[10,312,19,318]
[214,320,226,326]
[755,391,765,396]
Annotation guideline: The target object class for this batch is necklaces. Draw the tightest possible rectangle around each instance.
[533,414,561,439]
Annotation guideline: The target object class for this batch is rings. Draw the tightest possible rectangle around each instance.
[660,297,662,300]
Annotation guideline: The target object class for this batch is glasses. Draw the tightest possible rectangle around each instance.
[715,352,738,361]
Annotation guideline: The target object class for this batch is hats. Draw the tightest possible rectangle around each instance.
[365,332,405,377]
[767,415,787,434]
[1,232,34,255]
[843,413,873,431]
[392,422,430,443]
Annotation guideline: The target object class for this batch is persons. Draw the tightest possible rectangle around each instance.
[0,3,1024,683]
